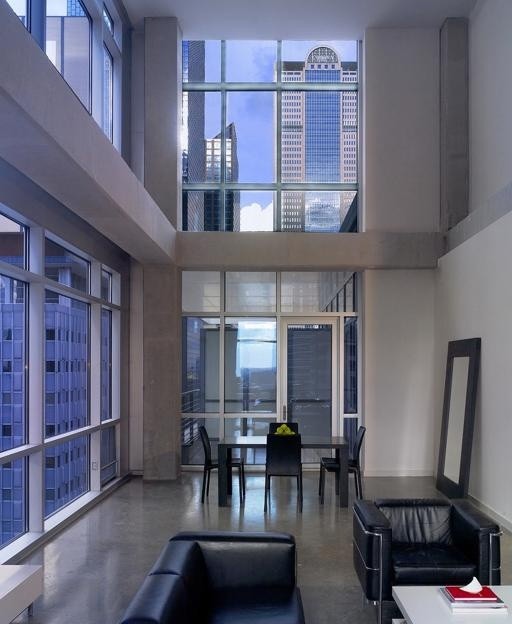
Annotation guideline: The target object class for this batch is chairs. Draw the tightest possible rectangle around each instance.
[265,435,302,515]
[198,423,245,503]
[269,423,298,435]
[319,425,368,503]
[353,496,501,622]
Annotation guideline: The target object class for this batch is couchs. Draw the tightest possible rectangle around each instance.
[120,528,306,623]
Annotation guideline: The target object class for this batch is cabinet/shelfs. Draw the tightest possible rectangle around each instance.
[1,563,45,622]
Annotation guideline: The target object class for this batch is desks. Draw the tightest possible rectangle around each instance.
[216,433,350,509]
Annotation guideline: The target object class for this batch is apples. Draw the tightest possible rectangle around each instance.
[276,424,296,434]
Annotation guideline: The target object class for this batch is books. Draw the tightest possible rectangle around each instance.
[438,585,510,616]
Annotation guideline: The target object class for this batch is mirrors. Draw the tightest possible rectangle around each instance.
[436,337,481,498]
